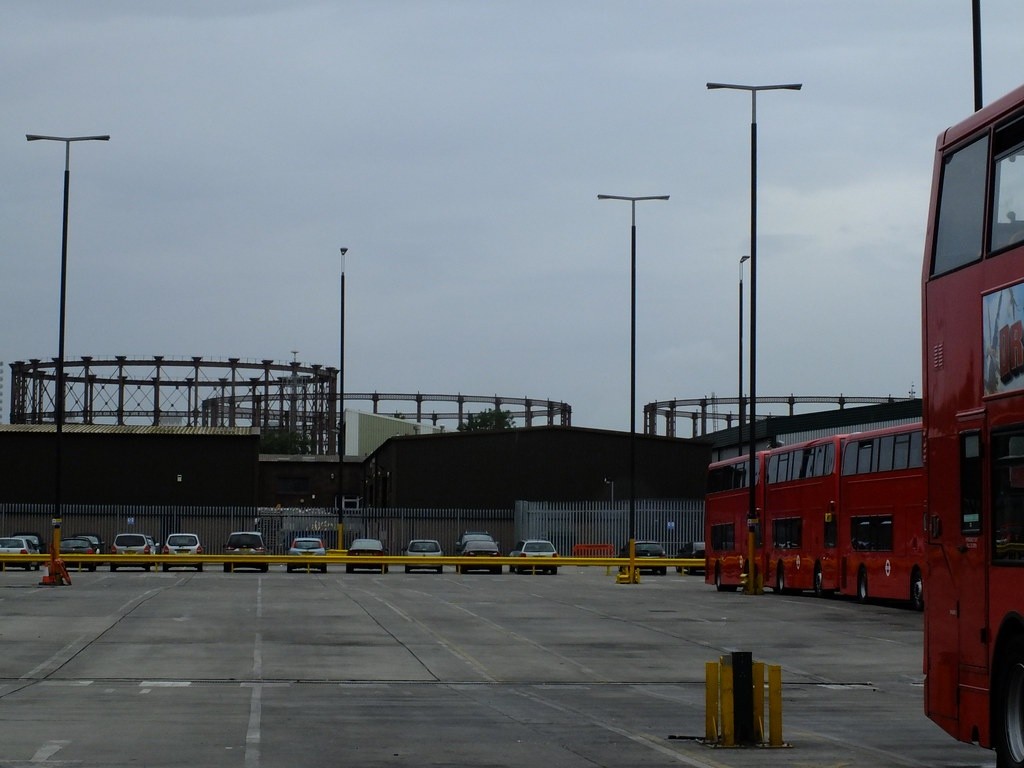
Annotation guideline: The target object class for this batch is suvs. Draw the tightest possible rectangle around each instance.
[509,538,562,575]
[456,531,500,550]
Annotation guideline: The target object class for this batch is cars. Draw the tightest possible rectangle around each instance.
[109,534,160,573]
[455,541,503,576]
[161,534,207,572]
[617,540,668,577]
[346,539,389,574]
[401,539,445,573]
[0,537,41,572]
[674,542,706,576]
[284,537,329,573]
[58,537,105,573]
[11,535,46,567]
[223,531,270,573]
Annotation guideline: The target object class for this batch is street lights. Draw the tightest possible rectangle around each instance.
[596,194,670,584]
[739,254,752,458]
[25,133,110,585]
[707,81,802,595]
[339,245,347,550]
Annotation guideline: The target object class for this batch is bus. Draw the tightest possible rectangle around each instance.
[701,83,1024,768]
